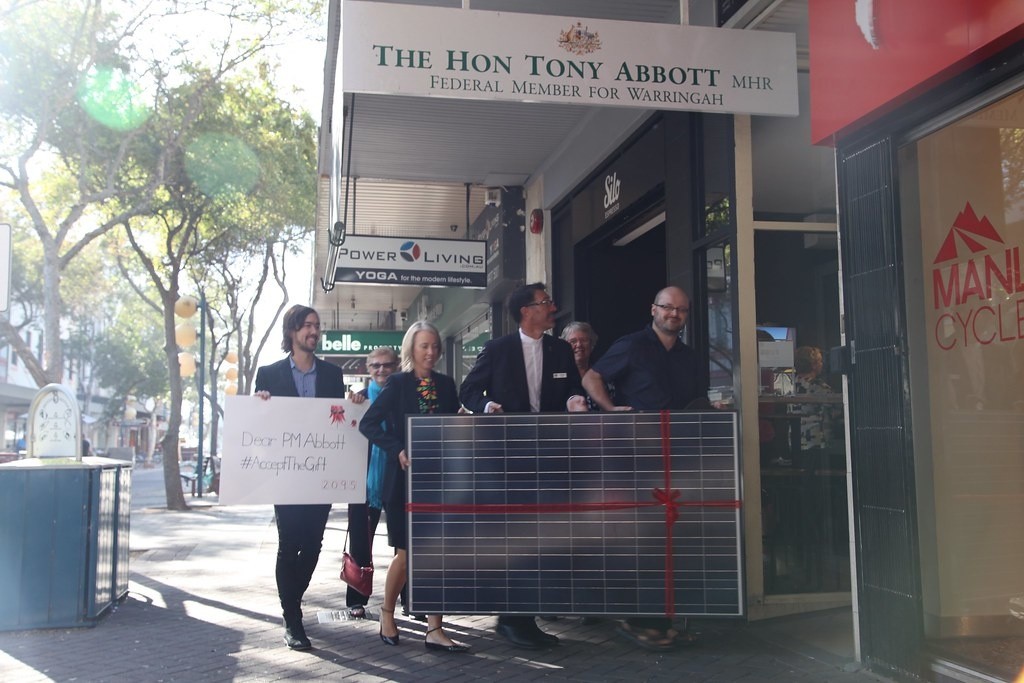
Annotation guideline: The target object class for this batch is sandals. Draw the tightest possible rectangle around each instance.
[661,626,698,646]
[616,620,675,653]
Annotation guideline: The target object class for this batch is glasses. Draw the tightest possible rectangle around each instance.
[526,299,555,307]
[369,362,395,369]
[655,303,689,314]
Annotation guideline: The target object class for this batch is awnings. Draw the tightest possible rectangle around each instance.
[0,381,41,406]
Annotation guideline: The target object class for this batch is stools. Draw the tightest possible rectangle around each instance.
[810,468,849,591]
[761,466,808,590]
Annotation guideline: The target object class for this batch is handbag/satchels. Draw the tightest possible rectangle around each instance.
[339,553,373,596]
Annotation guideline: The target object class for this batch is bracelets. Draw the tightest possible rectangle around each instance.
[567,395,579,401]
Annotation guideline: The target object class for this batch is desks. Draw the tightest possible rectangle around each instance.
[755,393,842,592]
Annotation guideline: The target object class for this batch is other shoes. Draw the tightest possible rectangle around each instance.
[284,632,310,650]
[380,608,399,645]
[348,604,365,617]
[424,626,469,651]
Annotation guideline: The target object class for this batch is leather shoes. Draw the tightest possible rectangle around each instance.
[497,616,561,649]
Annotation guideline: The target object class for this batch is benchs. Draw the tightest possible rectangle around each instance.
[180,455,211,498]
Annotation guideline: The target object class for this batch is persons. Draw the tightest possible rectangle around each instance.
[344,345,425,617]
[581,285,727,654]
[16,435,26,455]
[359,319,471,653]
[82,434,90,455]
[756,329,834,593]
[786,344,844,468]
[458,282,589,651]
[537,319,603,625]
[254,304,365,650]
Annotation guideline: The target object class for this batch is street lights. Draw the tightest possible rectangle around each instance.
[173,292,207,498]
